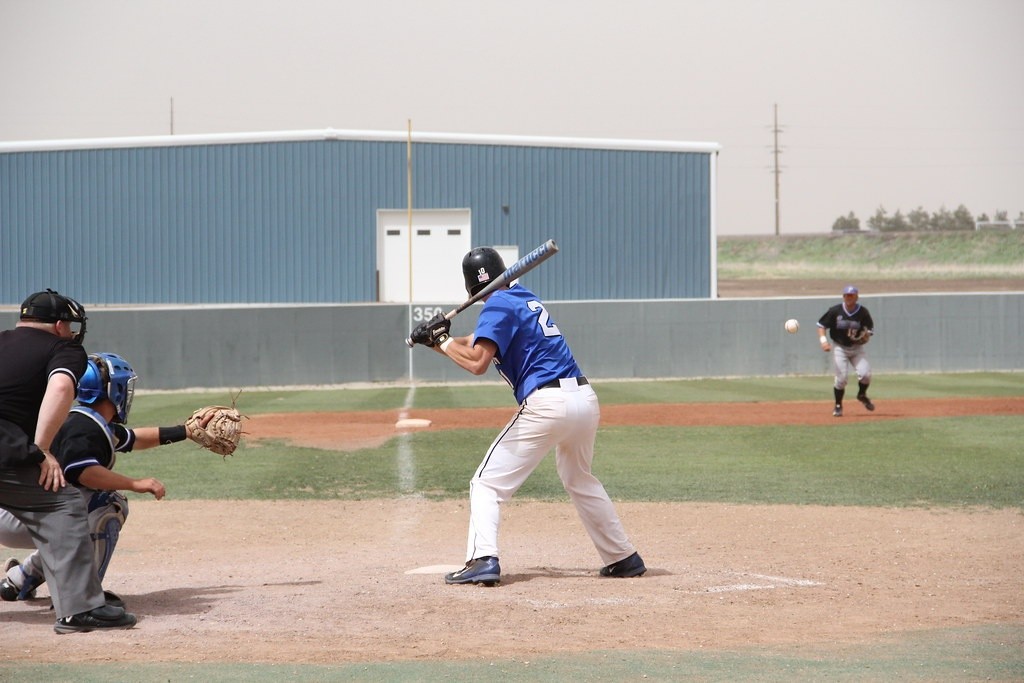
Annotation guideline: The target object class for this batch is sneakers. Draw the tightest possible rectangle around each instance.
[54,610,137,634]
[600,552,647,577]
[445,556,500,585]
[0,577,36,600]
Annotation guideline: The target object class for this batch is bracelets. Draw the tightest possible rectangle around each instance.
[819,336,827,344]
[159,424,186,445]
[440,336,454,352]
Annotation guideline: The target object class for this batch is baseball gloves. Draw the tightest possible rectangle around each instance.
[184,405,244,457]
[847,326,870,346]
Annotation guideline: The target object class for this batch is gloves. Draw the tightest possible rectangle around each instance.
[410,323,436,350]
[426,311,451,348]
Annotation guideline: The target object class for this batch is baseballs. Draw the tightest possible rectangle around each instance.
[784,318,800,334]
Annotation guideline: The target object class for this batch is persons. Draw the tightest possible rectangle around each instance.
[0,287,136,633]
[411,247,647,584]
[816,287,874,416]
[1,352,242,607]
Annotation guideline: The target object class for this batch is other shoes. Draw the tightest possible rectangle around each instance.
[832,403,843,416]
[857,395,875,411]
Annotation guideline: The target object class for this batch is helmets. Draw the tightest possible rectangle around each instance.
[462,248,509,299]
[75,351,138,425]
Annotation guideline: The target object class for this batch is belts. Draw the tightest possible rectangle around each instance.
[538,377,589,389]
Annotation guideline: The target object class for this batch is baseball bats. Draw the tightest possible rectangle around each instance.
[404,238,559,348]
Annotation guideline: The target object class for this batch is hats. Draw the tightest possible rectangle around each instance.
[843,286,858,297]
[20,290,83,325]
